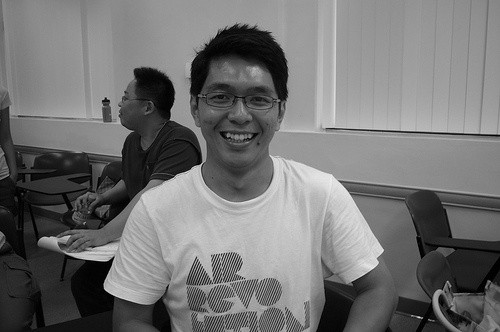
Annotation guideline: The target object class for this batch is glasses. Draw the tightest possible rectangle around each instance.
[122,96,151,101]
[198,91,281,110]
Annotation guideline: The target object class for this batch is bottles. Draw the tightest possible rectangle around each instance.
[70,195,96,226]
[102,97,112,122]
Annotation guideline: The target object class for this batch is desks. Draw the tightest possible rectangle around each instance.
[16,172,91,210]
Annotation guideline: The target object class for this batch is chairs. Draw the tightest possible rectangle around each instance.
[403,189,500,332]
[0,205,46,329]
[18,150,94,246]
[59,160,124,282]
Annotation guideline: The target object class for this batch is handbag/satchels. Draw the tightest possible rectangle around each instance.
[432,279,500,332]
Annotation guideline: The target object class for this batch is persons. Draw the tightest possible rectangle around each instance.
[1,86,26,258]
[56,23,399,332]
